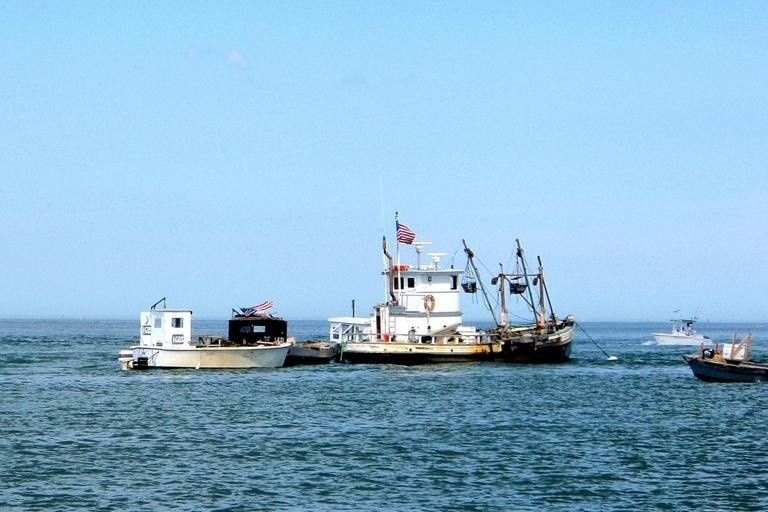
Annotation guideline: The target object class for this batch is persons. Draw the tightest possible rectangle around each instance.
[390,334,399,342]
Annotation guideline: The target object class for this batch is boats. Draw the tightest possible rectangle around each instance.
[652,319,768,382]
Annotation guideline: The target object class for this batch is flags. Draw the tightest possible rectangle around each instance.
[395,219,416,246]
[239,299,275,316]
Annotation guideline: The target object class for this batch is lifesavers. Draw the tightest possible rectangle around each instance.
[423,294,435,312]
